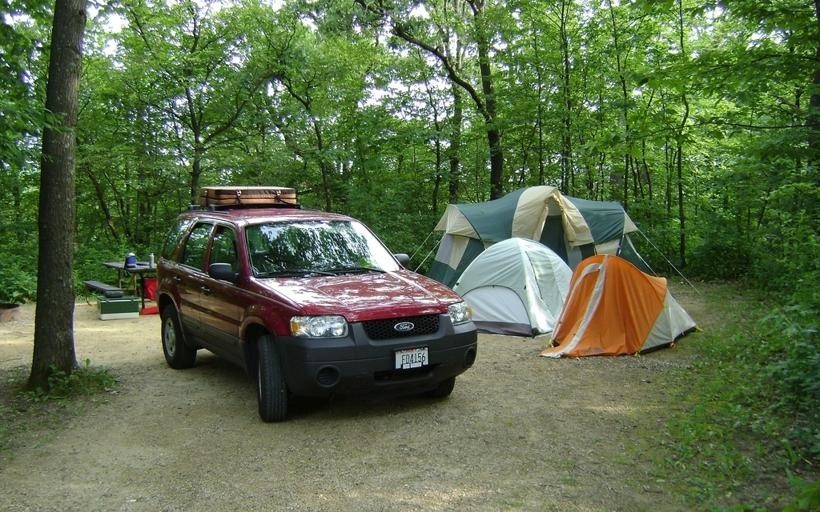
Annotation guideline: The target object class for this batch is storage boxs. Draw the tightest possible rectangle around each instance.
[98,298,139,320]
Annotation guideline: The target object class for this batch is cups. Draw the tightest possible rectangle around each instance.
[148,254,155,268]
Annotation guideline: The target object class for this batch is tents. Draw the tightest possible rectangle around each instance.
[538,253,703,359]
[427,184,659,290]
[451,236,573,339]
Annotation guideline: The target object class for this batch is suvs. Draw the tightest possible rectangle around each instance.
[156,204,477,422]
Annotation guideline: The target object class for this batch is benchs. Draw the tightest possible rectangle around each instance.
[83,281,124,309]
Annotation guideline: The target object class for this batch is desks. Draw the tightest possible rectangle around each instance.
[103,262,157,310]
